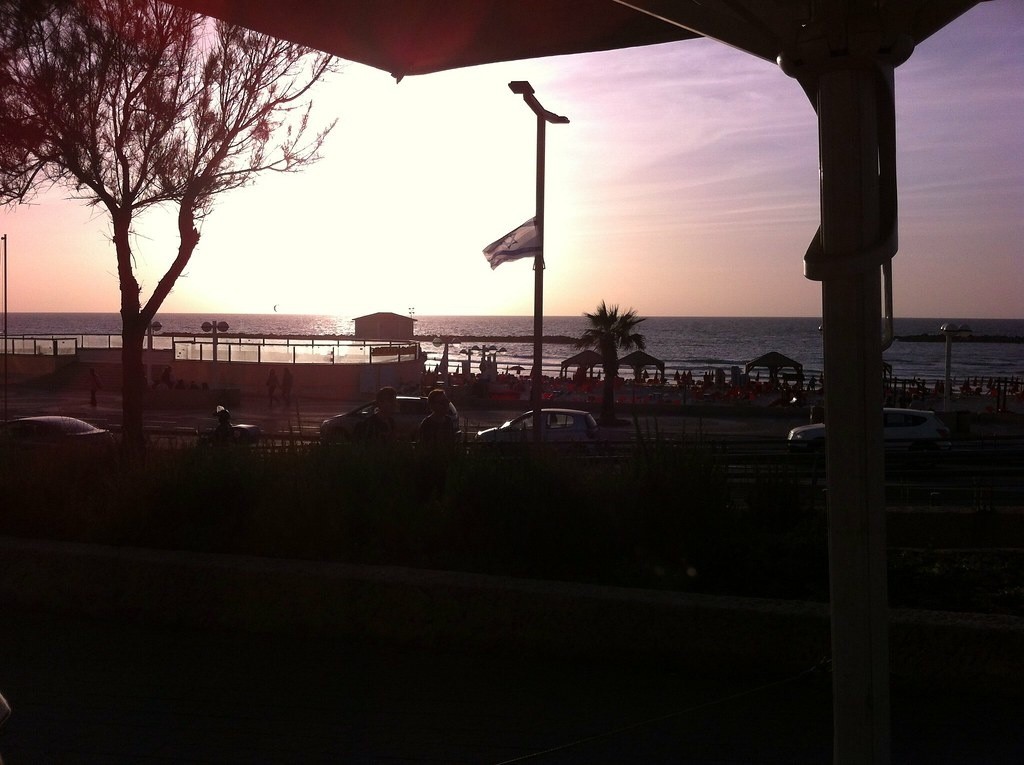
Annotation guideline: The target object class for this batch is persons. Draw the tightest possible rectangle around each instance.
[416,389,457,442]
[496,368,1021,414]
[266,368,280,406]
[353,387,398,442]
[281,367,293,408]
[89,367,101,406]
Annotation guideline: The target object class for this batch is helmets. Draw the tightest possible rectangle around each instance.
[214,405,231,423]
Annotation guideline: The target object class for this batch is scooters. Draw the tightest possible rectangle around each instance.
[195,426,253,471]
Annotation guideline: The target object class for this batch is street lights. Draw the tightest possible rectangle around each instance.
[433,336,462,389]
[459,344,508,375]
[940,321,971,417]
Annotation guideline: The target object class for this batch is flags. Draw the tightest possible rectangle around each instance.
[483,215,543,271]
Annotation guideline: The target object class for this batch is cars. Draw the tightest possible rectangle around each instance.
[475,406,603,454]
[0,416,115,461]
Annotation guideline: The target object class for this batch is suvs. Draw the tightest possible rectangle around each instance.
[318,393,461,449]
[787,406,952,466]
